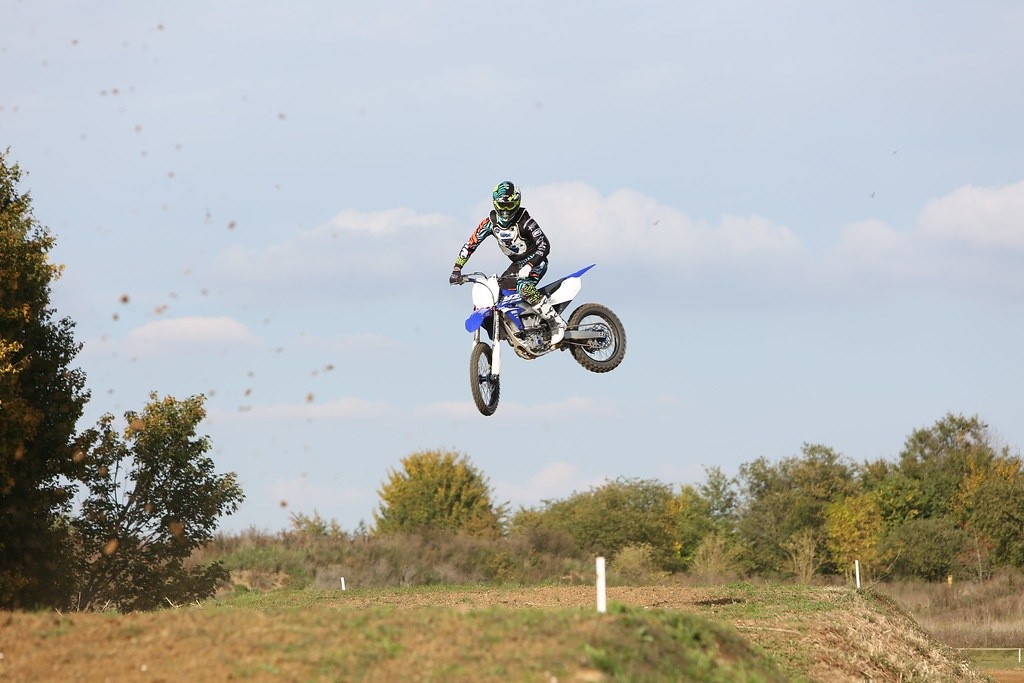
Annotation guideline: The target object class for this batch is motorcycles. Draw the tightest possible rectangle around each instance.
[450,264,627,416]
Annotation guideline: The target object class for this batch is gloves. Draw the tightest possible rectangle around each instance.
[449,271,461,284]
[519,263,532,280]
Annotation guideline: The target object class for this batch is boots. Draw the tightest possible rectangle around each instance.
[531,294,568,345]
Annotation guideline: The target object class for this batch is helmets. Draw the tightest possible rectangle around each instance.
[492,180,522,223]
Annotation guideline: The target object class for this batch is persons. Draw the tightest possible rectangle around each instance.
[449,182,569,346]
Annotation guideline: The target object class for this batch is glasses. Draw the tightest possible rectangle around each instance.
[493,200,519,211]
